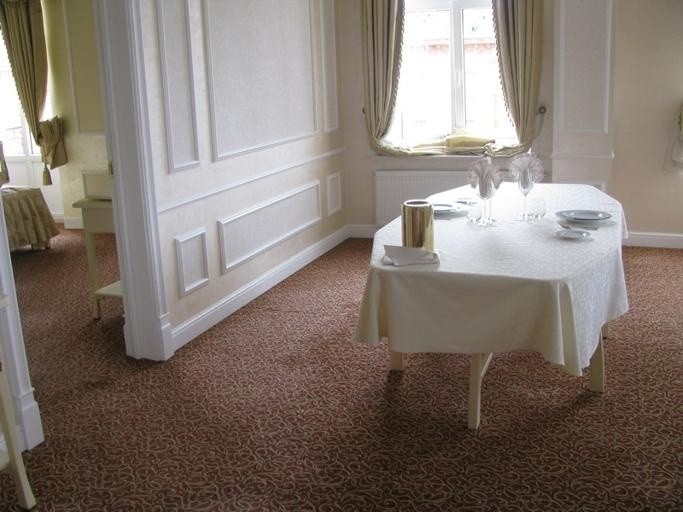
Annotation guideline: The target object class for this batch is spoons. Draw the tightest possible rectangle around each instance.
[559,224,598,231]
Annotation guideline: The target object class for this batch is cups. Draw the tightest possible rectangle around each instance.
[526,197,546,224]
[465,201,482,225]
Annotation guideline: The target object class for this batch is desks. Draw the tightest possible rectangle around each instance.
[72,197,123,322]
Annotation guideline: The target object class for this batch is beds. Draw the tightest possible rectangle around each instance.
[3,182,61,253]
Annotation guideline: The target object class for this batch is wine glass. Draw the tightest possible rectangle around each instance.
[477,176,496,226]
[516,170,534,221]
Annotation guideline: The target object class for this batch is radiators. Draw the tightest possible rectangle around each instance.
[375,169,545,229]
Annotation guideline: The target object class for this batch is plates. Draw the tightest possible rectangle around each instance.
[454,194,477,204]
[557,230,589,241]
[431,201,456,214]
[555,210,613,222]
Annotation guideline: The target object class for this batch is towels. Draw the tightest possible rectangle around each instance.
[509,153,544,189]
[382,244,440,266]
[469,161,503,196]
